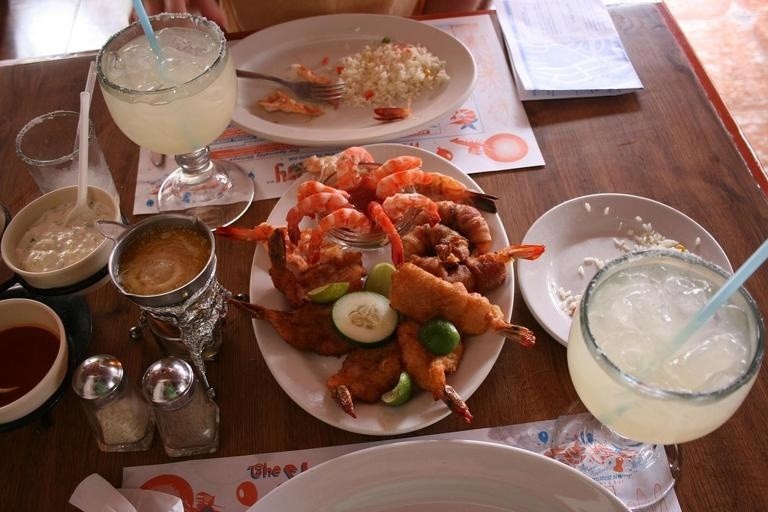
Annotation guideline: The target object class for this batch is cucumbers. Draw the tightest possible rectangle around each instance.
[331,291,399,347]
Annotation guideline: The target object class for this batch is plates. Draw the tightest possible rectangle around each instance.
[247,141,517,438]
[229,15,477,148]
[247,439,631,512]
[516,194,740,346]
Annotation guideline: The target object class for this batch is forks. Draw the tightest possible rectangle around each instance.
[233,66,345,104]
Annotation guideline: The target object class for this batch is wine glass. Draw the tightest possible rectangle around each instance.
[96,15,253,231]
[556,247,763,511]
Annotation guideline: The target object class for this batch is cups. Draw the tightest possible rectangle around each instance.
[15,109,121,209]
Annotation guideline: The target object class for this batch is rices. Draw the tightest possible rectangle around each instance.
[555,199,702,316]
[332,26,450,111]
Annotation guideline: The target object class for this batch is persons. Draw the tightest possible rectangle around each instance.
[127,0,494,41]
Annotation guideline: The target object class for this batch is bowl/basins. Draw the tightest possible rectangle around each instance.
[4,184,123,299]
[1,300,70,427]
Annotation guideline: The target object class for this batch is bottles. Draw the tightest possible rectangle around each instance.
[76,356,226,460]
